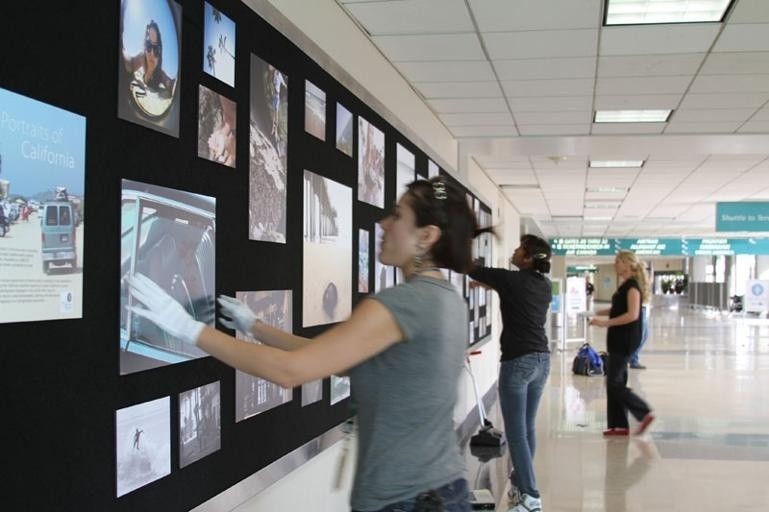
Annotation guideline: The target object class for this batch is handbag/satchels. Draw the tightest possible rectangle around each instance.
[573,343,593,376]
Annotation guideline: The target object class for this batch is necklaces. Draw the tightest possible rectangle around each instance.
[402,267,445,281]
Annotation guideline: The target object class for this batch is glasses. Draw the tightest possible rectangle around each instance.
[144,40,160,56]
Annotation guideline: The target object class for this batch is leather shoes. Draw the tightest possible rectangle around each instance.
[639,417,652,434]
[603,430,630,436]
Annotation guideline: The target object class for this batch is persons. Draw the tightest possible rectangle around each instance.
[265,66,287,142]
[121,17,176,95]
[580,276,597,320]
[586,248,657,438]
[467,231,556,511]
[125,174,502,511]
[627,257,653,369]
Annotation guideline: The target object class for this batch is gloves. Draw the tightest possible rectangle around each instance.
[125,272,204,348]
[217,294,262,340]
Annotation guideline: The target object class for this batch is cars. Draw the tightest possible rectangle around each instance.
[121,191,215,362]
[37,200,81,274]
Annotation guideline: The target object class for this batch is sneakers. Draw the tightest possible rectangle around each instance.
[508,486,519,502]
[508,495,543,512]
